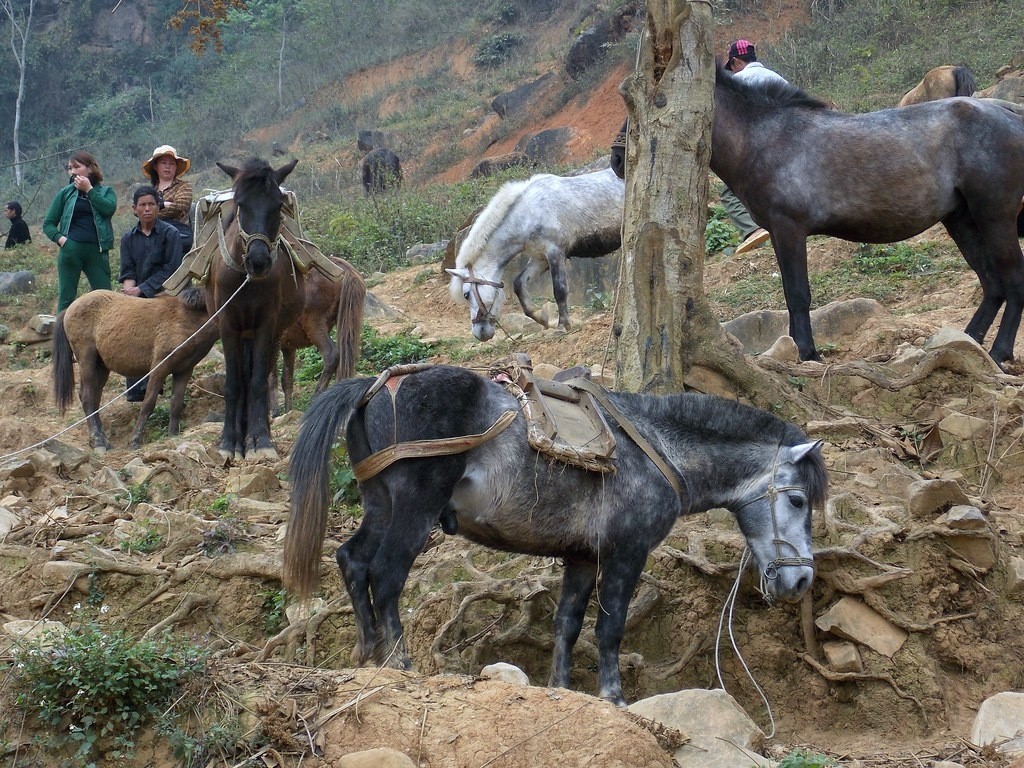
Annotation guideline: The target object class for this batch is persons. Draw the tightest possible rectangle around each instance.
[42,151,118,313]
[142,145,192,261]
[4,202,32,250]
[117,186,181,402]
[725,40,789,253]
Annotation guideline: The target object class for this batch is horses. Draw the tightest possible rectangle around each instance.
[282,364,828,709]
[445,167,625,343]
[610,63,1024,370]
[54,156,366,463]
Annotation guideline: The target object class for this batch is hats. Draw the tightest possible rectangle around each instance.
[723,40,756,70]
[142,144,191,180]
